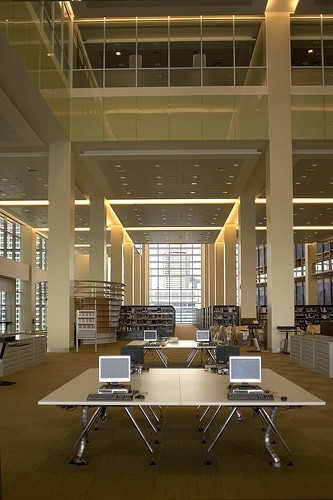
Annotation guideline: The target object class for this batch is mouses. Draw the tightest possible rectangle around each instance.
[138,394,144,399]
[281,396,287,401]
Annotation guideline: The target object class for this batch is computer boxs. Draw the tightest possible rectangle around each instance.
[199,342,210,346]
[98,385,131,394]
[130,365,143,375]
[217,366,229,374]
[228,385,264,394]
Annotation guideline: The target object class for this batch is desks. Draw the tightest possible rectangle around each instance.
[38,340,326,467]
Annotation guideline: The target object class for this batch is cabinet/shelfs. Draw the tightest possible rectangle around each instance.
[197,306,333,379]
[76,310,97,351]
[121,306,175,337]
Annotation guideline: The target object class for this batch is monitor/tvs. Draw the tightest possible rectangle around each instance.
[320,321,333,336]
[216,346,240,364]
[98,356,131,388]
[229,356,264,389]
[121,345,144,364]
[144,329,157,341]
[196,330,210,341]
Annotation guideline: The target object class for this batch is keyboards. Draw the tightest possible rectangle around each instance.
[228,392,274,400]
[86,394,133,401]
